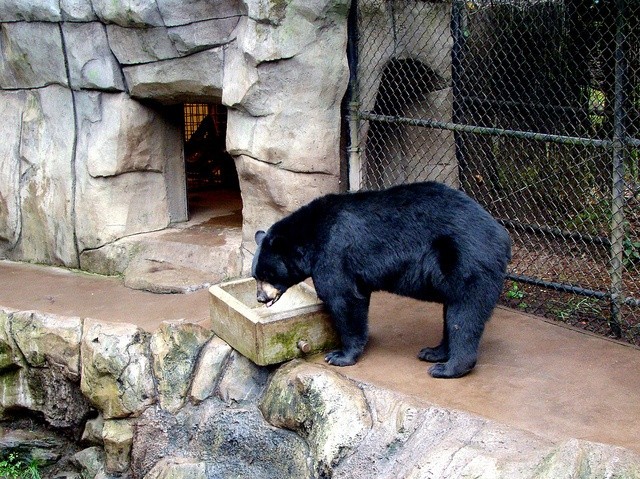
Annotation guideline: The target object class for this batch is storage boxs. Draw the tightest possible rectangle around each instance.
[209,279,334,367]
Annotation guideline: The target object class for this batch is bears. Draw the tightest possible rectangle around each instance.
[251,181,512,379]
